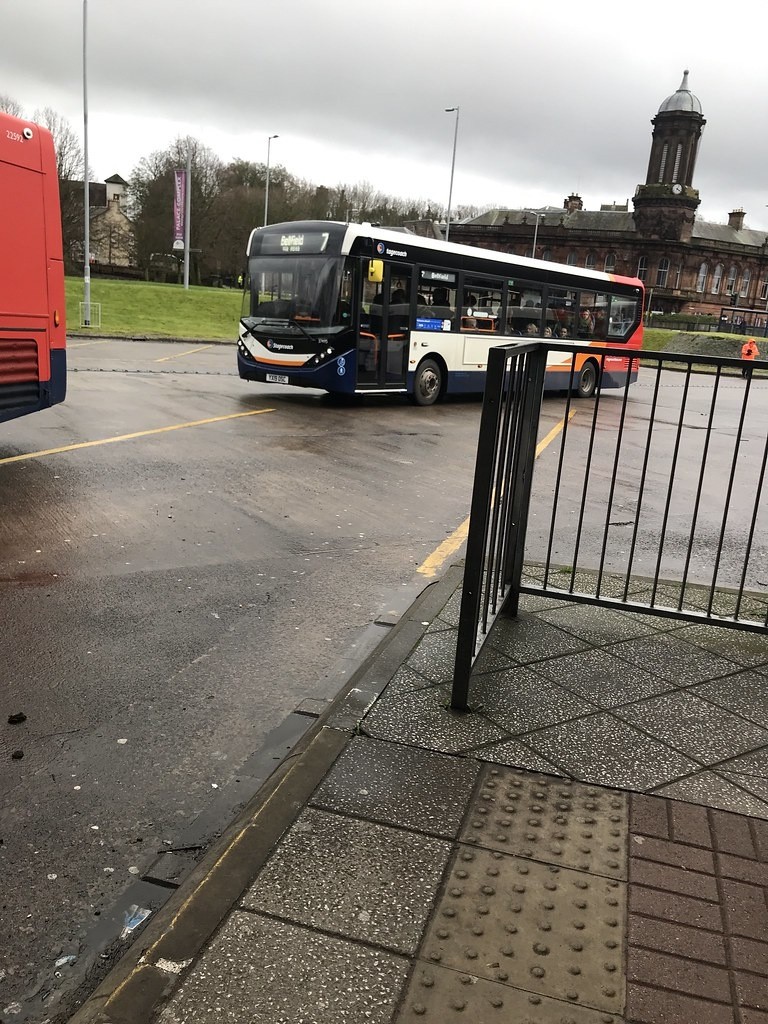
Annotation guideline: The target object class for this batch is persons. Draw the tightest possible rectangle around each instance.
[274,272,632,340]
[742,339,760,378]
[656,303,767,335]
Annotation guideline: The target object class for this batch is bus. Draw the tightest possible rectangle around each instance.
[236,219,646,407]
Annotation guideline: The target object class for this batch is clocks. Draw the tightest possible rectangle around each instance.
[671,183,685,195]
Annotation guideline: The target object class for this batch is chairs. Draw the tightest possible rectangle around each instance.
[514,314,595,338]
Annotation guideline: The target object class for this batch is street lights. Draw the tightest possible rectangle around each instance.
[264,134,279,226]
[530,211,546,259]
[445,105,460,241]
